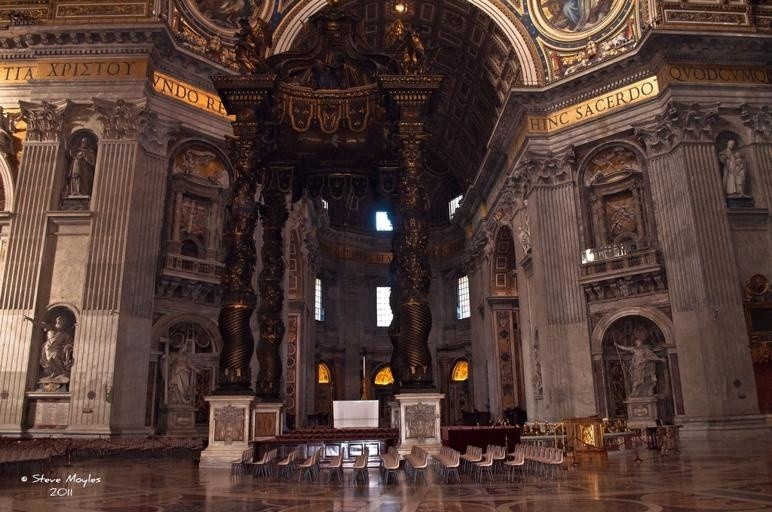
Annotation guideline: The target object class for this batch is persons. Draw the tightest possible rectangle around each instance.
[719,139,746,198]
[23,314,73,392]
[70,137,95,196]
[614,339,666,397]
[400,20,429,76]
[234,18,266,74]
[170,343,201,404]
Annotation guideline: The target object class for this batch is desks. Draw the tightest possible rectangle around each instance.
[441,425,521,467]
[646,425,682,450]
[604,431,635,449]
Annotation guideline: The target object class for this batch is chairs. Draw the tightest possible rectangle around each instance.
[230,443,565,489]
